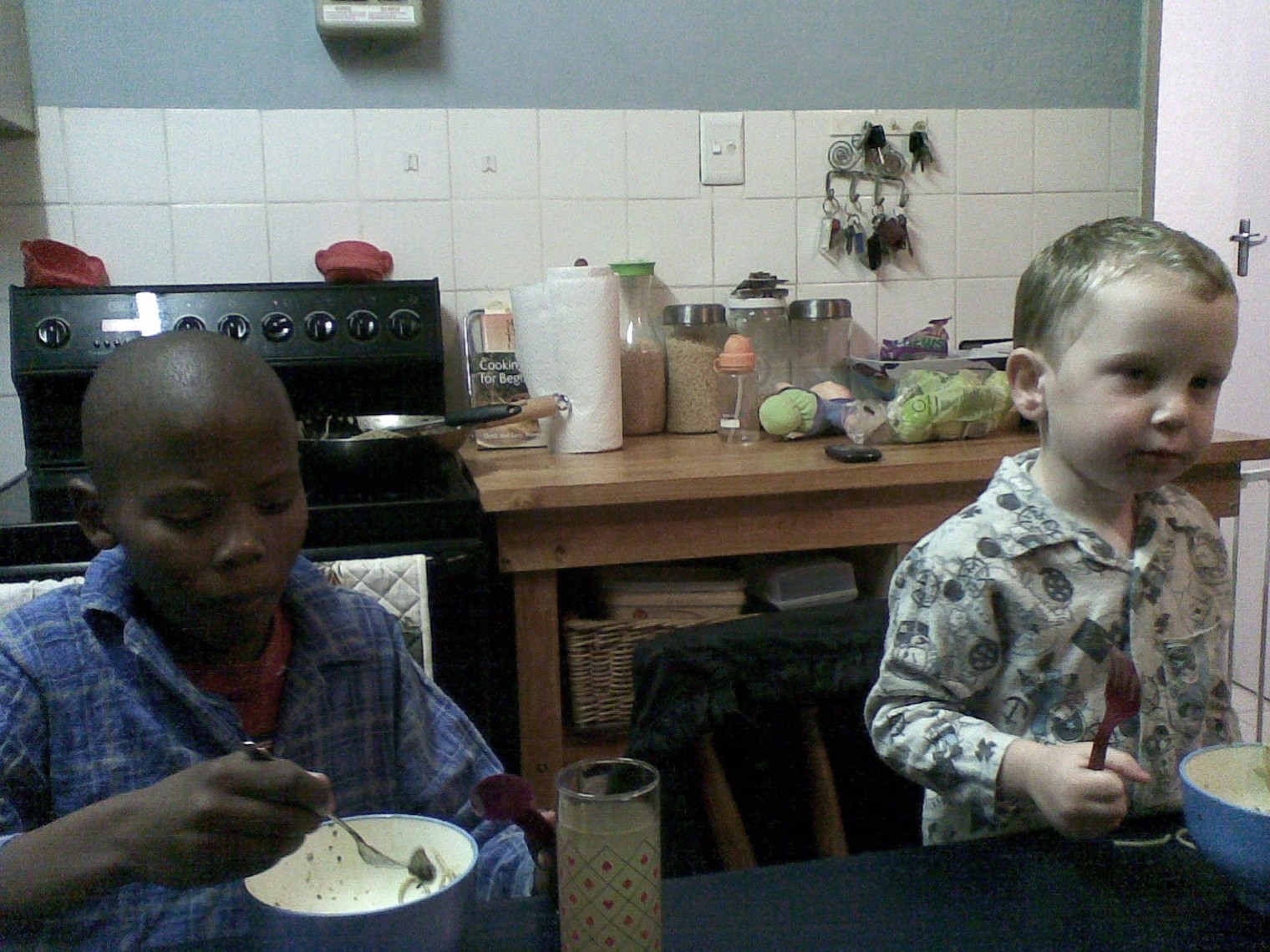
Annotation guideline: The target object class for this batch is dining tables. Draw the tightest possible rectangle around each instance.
[140,820,1270,950]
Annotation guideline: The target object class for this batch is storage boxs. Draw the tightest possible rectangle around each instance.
[562,550,860,735]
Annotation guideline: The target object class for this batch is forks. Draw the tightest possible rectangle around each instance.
[1084,659,1139,770]
[243,739,410,870]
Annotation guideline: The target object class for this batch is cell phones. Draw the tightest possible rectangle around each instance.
[825,442,884,464]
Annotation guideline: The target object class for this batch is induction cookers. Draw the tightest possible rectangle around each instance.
[0,276,479,551]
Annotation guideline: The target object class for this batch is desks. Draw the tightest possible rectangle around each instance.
[454,429,1270,833]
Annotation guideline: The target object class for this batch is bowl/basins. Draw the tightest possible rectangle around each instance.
[1177,740,1270,919]
[233,814,481,952]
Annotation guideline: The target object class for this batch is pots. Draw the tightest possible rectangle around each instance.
[293,391,570,477]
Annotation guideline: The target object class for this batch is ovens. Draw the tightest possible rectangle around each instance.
[0,513,504,751]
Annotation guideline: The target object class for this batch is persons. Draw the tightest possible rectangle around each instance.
[858,212,1249,848]
[0,330,558,951]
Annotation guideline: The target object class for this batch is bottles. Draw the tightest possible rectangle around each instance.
[605,255,856,445]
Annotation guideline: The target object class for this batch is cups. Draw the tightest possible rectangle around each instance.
[556,756,665,952]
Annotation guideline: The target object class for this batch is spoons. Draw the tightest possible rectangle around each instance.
[475,773,556,868]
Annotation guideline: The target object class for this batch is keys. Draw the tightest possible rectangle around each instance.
[818,121,935,273]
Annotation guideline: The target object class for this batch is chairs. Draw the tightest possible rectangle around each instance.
[629,590,950,872]
